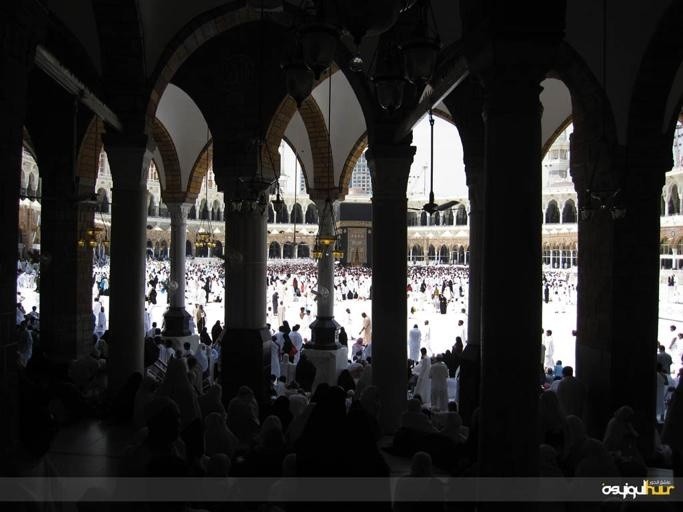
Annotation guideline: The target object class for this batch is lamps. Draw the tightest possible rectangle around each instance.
[309,63,345,259]
[74,97,108,248]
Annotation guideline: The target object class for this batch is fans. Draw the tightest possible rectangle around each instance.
[407,97,467,213]
